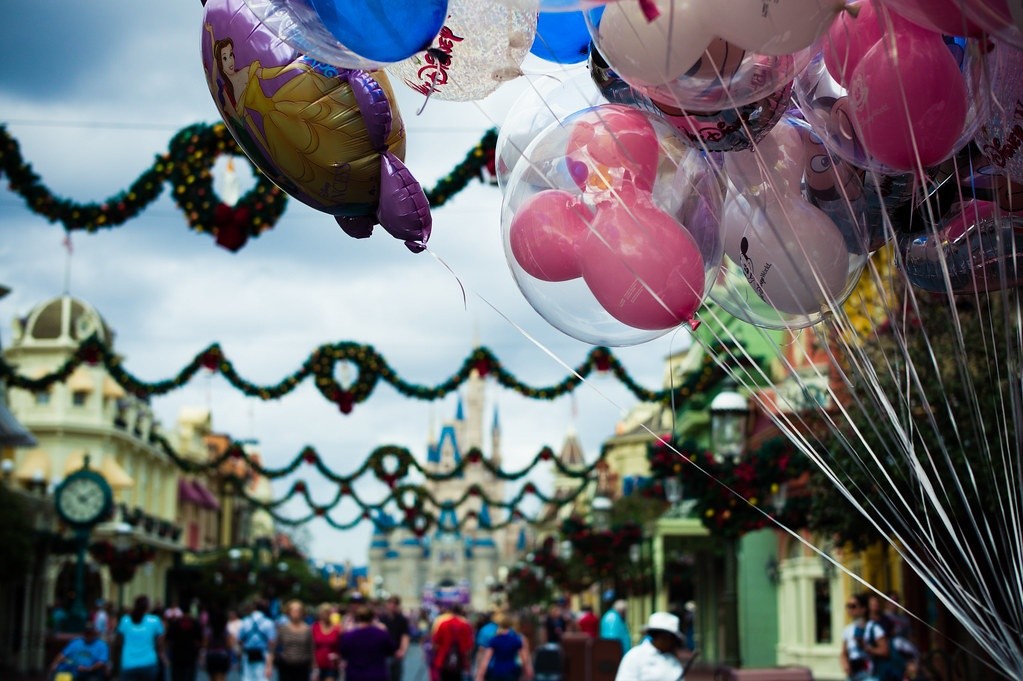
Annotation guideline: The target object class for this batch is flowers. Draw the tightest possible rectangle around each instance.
[1,329,763,407]
[486,434,822,612]
[150,433,600,539]
[1,122,510,253]
[90,540,156,585]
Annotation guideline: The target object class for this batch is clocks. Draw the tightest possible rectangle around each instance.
[59,477,105,523]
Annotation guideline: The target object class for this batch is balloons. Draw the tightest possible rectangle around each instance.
[203,0,1022,348]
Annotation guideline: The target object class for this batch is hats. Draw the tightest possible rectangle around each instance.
[641,612,684,642]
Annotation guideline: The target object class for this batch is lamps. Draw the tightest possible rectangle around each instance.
[709,375,749,465]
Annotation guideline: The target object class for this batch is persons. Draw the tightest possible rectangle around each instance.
[671,602,695,651]
[547,597,599,643]
[235,594,411,681]
[842,591,918,681]
[416,601,547,681]
[48,591,240,681]
[599,599,630,656]
[614,612,689,681]
